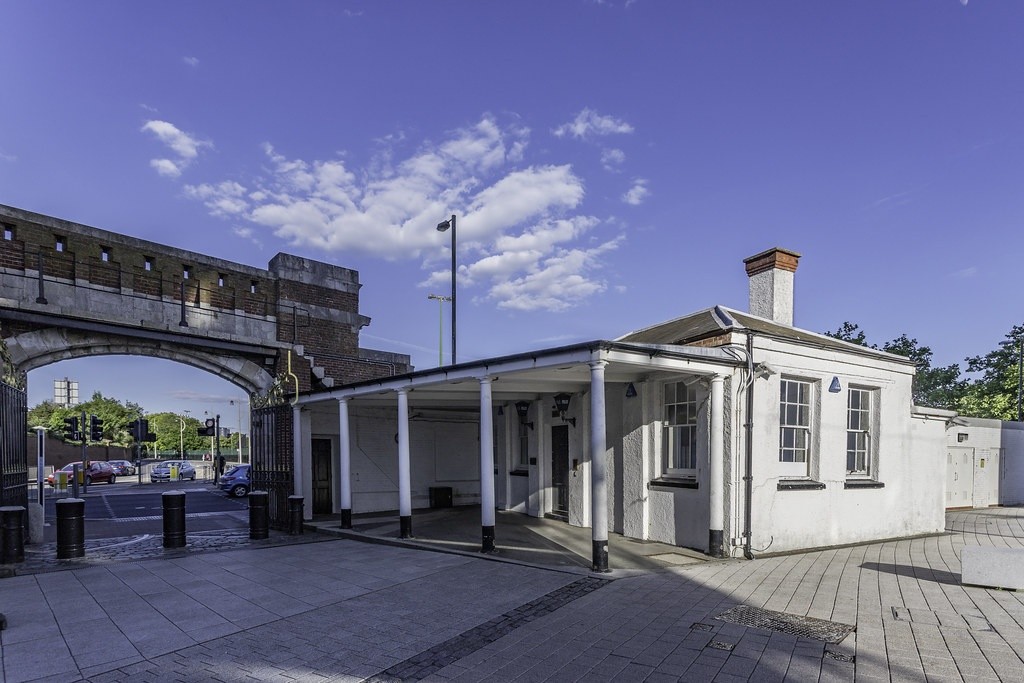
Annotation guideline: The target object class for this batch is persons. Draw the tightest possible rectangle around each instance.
[212,451,226,486]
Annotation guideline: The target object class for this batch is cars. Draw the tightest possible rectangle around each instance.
[150,460,197,483]
[218,464,252,498]
[48,460,116,487]
[108,460,136,475]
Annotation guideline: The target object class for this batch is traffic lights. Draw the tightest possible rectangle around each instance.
[64,417,77,440]
[90,415,104,442]
[206,418,214,435]
[126,420,139,442]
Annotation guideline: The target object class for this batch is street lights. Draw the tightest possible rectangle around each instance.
[149,417,158,460]
[436,215,456,364]
[174,413,186,458]
[230,399,242,464]
[428,295,452,367]
